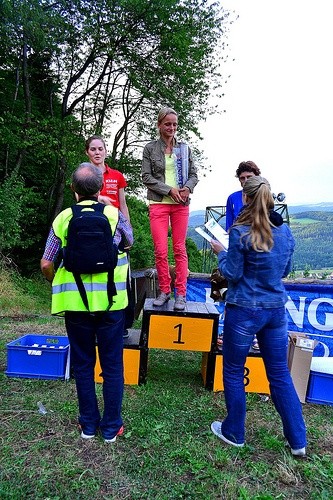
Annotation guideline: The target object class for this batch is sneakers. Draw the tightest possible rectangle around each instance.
[104,425,123,442]
[285,438,306,455]
[79,424,95,439]
[211,421,244,447]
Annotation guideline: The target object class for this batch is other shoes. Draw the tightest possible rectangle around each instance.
[123,328,129,338]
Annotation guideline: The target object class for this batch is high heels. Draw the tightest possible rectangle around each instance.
[153,292,172,306]
[175,295,188,309]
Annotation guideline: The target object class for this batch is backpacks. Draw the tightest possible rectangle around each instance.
[63,206,118,275]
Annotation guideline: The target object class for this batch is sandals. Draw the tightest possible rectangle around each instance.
[251,337,260,350]
[216,332,226,347]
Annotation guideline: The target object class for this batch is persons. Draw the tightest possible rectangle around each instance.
[141,107,197,310]
[41,162,133,443]
[210,175,307,456]
[85,136,131,337]
[216,161,261,349]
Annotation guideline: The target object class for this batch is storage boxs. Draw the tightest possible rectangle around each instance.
[4,334,70,383]
[304,357,333,406]
[288,333,321,404]
